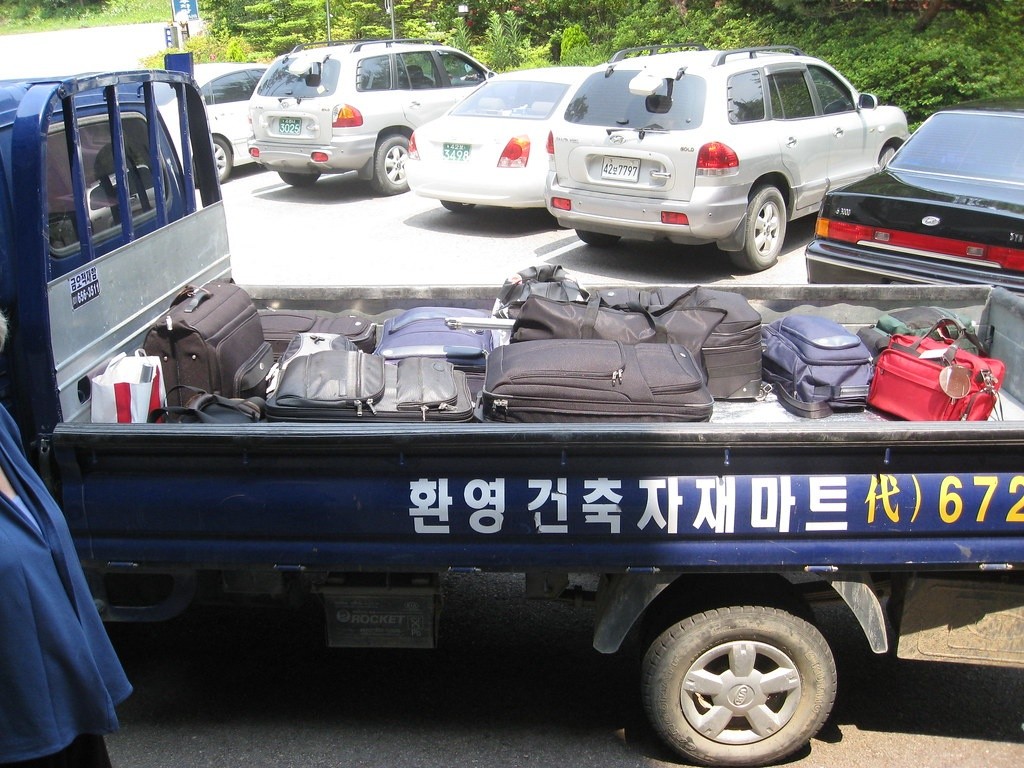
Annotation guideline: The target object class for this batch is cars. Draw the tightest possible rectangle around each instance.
[803,98,1024,295]
[404,63,594,214]
[154,61,300,191]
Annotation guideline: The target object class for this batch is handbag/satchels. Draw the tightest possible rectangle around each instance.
[866,316,1006,420]
[146,383,265,424]
[510,287,763,400]
[89,348,171,424]
[492,264,587,319]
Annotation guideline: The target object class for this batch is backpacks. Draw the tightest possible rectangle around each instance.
[266,332,360,398]
[856,306,990,367]
[761,314,875,420]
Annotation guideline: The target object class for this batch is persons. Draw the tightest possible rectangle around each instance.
[0,309,134,768]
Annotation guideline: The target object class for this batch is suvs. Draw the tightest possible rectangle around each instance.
[542,44,912,272]
[245,35,500,197]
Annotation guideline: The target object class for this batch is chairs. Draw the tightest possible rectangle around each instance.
[404,65,434,89]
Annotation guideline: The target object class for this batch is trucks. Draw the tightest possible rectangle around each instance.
[0,50,1024,768]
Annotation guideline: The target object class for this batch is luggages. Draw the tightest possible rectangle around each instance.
[257,307,515,424]
[143,279,274,407]
[481,338,715,424]
